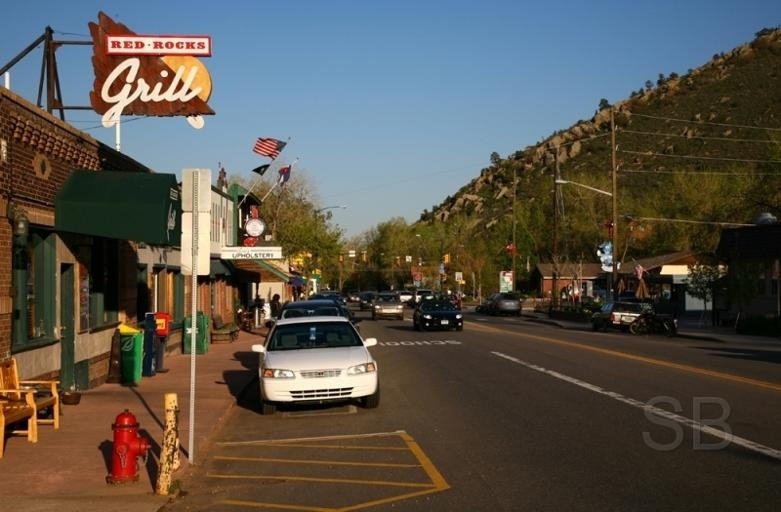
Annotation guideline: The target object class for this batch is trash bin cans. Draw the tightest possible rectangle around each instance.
[116,321,144,385]
[182,314,209,353]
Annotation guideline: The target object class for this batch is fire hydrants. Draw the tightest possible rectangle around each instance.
[107,407,153,485]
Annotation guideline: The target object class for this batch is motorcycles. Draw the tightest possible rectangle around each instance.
[474,301,498,317]
[628,306,680,337]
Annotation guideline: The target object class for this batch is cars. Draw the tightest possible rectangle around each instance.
[251,316,381,416]
[414,298,464,333]
[302,282,460,322]
[266,298,362,337]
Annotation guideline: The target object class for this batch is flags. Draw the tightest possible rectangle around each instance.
[631,258,643,280]
[252,136,289,162]
[278,164,292,186]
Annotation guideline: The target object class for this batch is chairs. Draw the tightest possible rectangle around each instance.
[0,389,34,462]
[0,359,59,442]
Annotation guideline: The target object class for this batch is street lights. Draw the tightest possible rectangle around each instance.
[414,233,459,279]
[310,205,348,292]
[553,178,619,300]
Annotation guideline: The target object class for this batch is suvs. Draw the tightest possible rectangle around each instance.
[484,291,524,317]
[590,299,645,331]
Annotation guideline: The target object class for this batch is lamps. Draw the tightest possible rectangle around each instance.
[4,195,31,240]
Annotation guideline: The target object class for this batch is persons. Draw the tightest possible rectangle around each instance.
[271,294,282,319]
